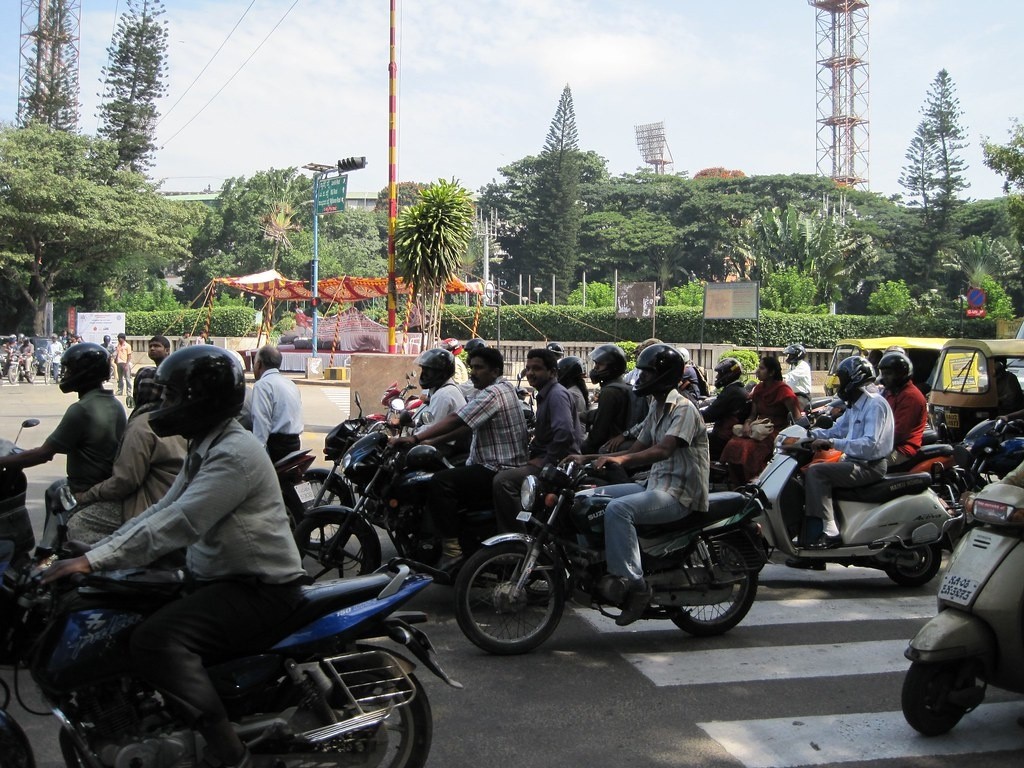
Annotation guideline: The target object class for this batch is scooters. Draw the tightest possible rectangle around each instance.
[902,445,1024,737]
[708,398,1024,586]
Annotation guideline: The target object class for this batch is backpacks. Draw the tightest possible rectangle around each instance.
[688,368,709,396]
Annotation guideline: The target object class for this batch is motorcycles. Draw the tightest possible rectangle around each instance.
[823,318,1024,443]
[294,368,776,656]
[0,419,463,768]
[8,349,37,384]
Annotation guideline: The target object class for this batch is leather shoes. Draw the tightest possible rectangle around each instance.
[785,554,826,571]
[805,533,842,551]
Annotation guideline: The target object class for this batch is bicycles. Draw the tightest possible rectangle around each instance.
[44,350,57,384]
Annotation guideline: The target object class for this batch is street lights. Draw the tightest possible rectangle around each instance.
[522,297,529,305]
[534,287,542,304]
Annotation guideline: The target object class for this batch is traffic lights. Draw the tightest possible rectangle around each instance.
[310,297,321,308]
[338,157,366,172]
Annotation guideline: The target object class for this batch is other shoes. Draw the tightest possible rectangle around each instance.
[616,581,654,625]
[197,742,251,767]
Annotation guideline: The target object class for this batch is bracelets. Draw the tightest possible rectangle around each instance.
[413,435,419,443]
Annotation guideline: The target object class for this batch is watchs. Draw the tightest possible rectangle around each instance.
[828,438,834,448]
[623,430,631,440]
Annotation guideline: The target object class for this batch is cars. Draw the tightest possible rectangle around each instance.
[29,338,64,375]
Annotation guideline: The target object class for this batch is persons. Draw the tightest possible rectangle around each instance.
[386,337,928,627]
[196,331,205,345]
[42,345,308,768]
[101,335,115,354]
[237,346,305,465]
[147,336,170,366]
[994,357,1024,424]
[0,343,189,556]
[0,329,84,384]
[178,331,191,349]
[113,333,132,397]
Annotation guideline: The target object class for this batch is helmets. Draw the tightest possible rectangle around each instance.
[836,356,876,401]
[713,357,742,389]
[417,348,456,388]
[633,344,684,398]
[59,344,111,393]
[546,344,564,354]
[557,357,583,384]
[675,345,692,363]
[879,352,913,390]
[147,345,245,436]
[405,444,444,474]
[590,345,627,384]
[783,344,807,366]
